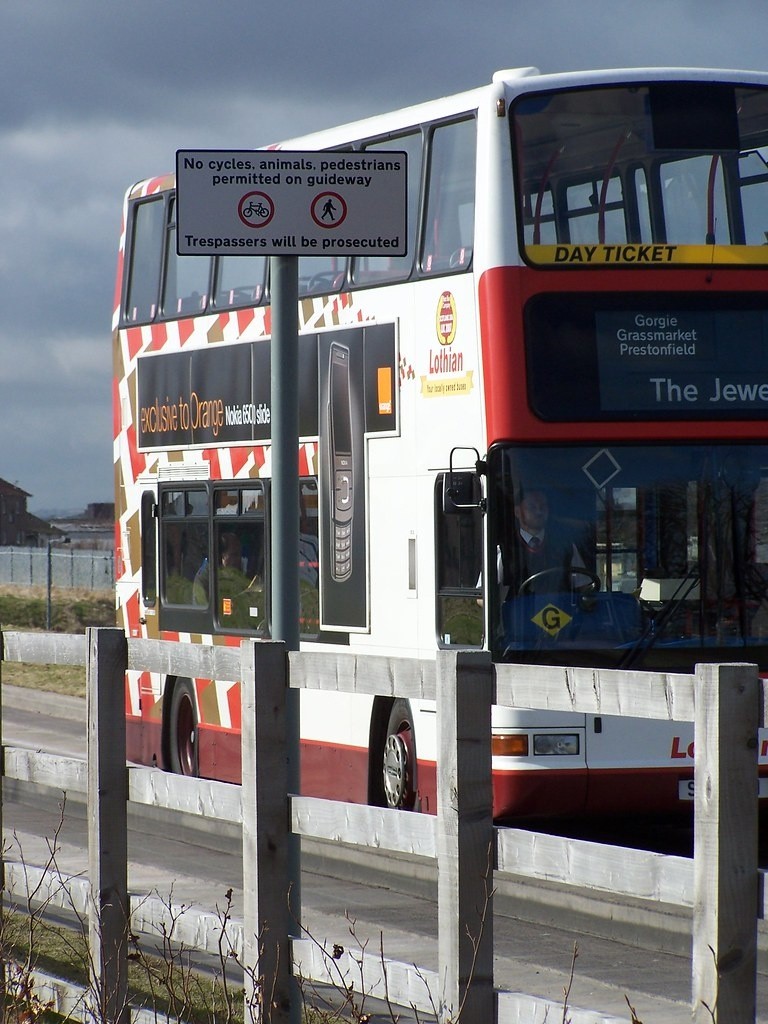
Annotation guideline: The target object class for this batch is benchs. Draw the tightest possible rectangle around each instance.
[332,270,405,289]
[228,286,258,305]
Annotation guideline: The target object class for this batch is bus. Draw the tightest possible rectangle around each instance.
[112,64,768,825]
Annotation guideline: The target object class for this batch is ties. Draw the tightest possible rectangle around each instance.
[531,536,540,550]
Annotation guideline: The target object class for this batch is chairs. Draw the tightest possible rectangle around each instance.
[474,484,597,575]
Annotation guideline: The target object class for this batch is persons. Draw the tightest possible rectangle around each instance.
[191,533,242,607]
[475,484,595,605]
